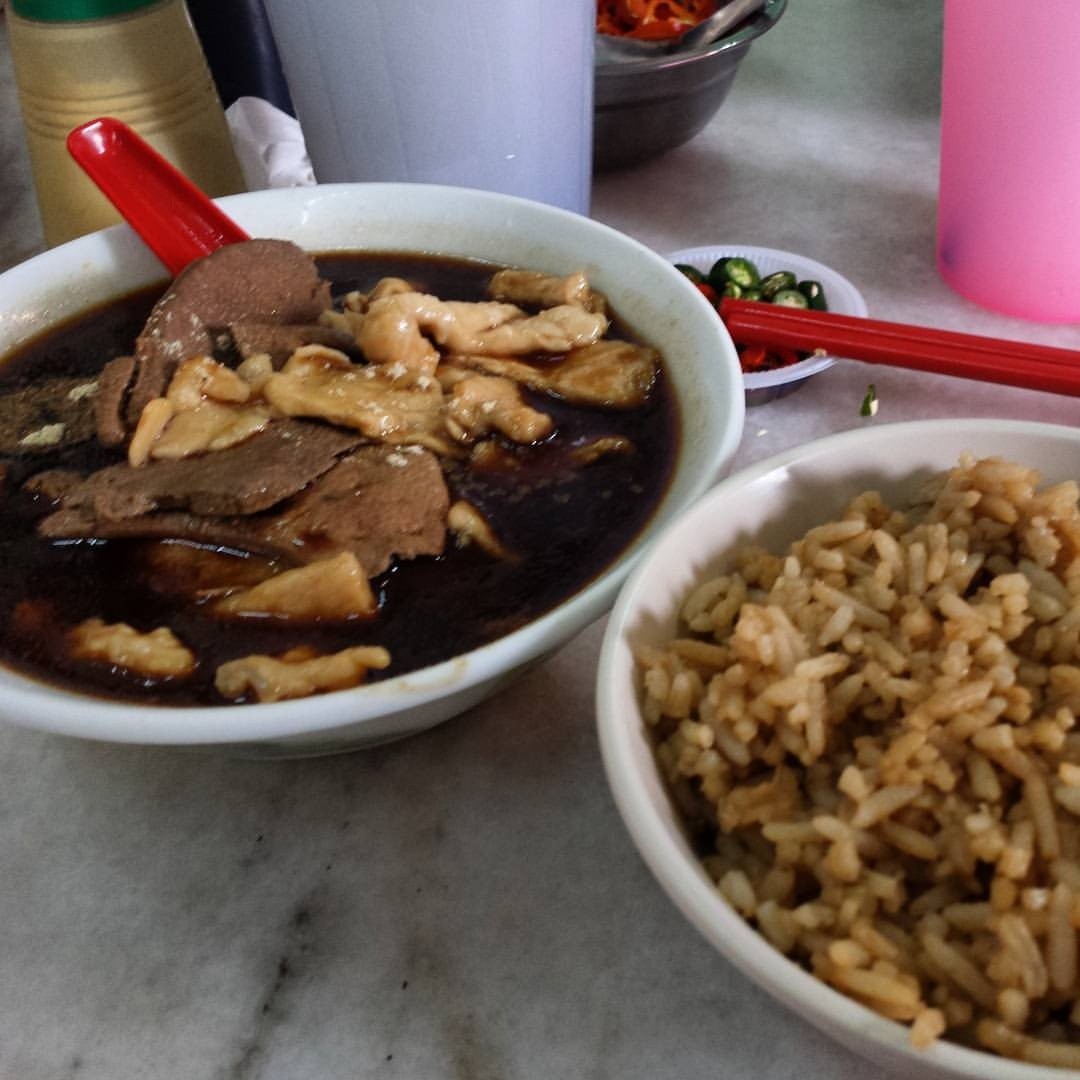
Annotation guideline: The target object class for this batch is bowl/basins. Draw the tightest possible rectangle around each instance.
[0,179,753,755]
[590,0,789,188]
[666,243,871,406]
[594,417,1080,1080]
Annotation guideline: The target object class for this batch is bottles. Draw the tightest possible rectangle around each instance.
[4,0,249,243]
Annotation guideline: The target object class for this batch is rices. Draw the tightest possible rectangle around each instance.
[636,439,1078,1070]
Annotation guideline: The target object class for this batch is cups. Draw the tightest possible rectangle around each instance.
[935,0,1078,326]
[267,0,594,215]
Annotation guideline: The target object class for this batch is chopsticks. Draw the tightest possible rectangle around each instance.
[718,295,1079,401]
